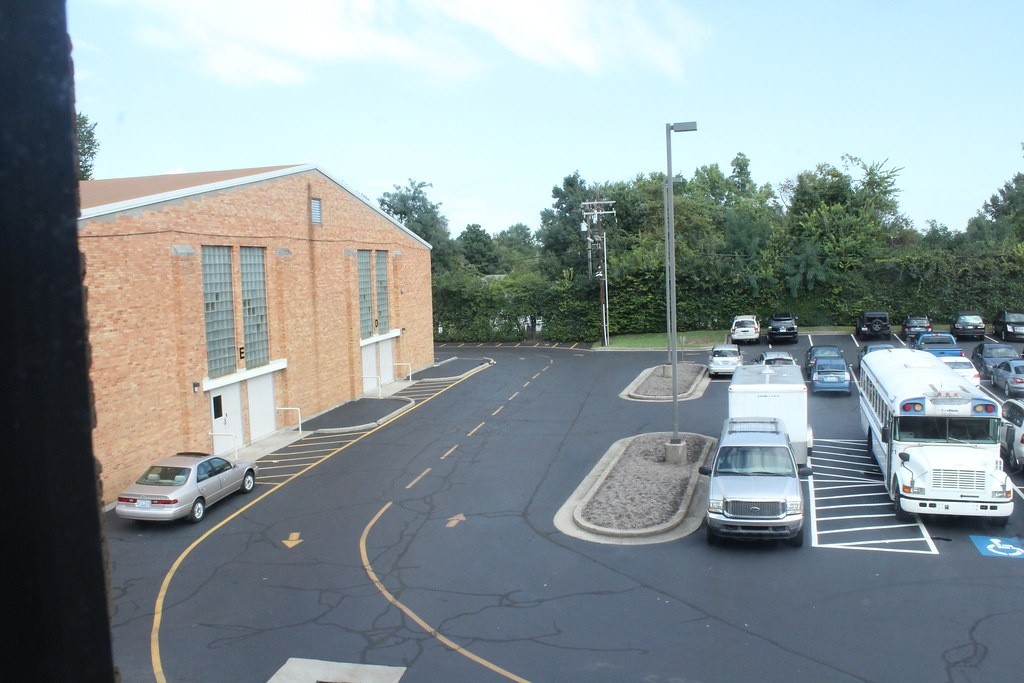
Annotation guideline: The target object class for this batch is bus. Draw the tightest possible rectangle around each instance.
[860,348,1015,527]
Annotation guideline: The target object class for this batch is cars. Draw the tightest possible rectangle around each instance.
[950,311,985,341]
[990,358,1024,398]
[751,351,797,366]
[938,356,980,389]
[971,343,1020,380]
[805,345,853,396]
[114,451,259,523]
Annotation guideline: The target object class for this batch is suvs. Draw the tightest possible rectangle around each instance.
[901,315,932,342]
[856,309,891,341]
[699,417,814,549]
[731,315,762,345]
[765,312,798,344]
[992,309,1024,341]
[707,345,745,380]
[1001,398,1024,474]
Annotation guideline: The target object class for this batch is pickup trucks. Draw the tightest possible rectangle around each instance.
[910,331,964,358]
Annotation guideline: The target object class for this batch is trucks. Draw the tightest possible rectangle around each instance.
[728,365,813,470]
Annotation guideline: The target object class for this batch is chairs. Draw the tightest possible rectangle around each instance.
[146,473,161,482]
[173,475,186,484]
[197,465,208,481]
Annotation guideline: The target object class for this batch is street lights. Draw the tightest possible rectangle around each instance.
[663,121,699,466]
[662,176,684,379]
